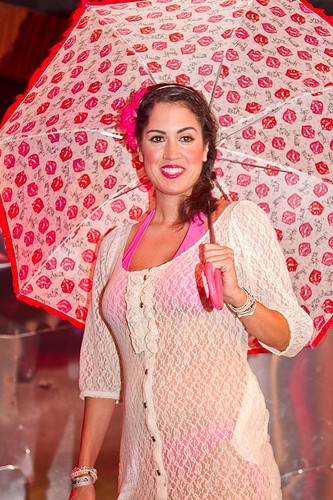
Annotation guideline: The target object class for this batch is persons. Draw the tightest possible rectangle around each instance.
[68,83,314,499]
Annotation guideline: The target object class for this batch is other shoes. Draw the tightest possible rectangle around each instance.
[17,362,35,383]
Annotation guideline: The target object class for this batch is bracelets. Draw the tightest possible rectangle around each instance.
[227,286,256,317]
[71,467,98,487]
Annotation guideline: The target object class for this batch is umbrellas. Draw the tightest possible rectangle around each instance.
[0,0,333,356]
